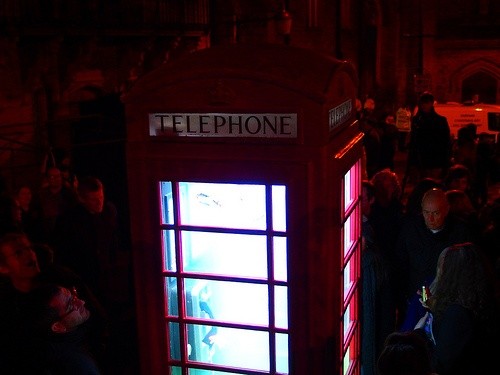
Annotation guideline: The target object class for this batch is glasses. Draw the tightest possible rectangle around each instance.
[59,287,78,318]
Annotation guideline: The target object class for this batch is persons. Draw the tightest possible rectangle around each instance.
[1,30,500,375]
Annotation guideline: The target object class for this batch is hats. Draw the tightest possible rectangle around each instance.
[419,92,433,103]
